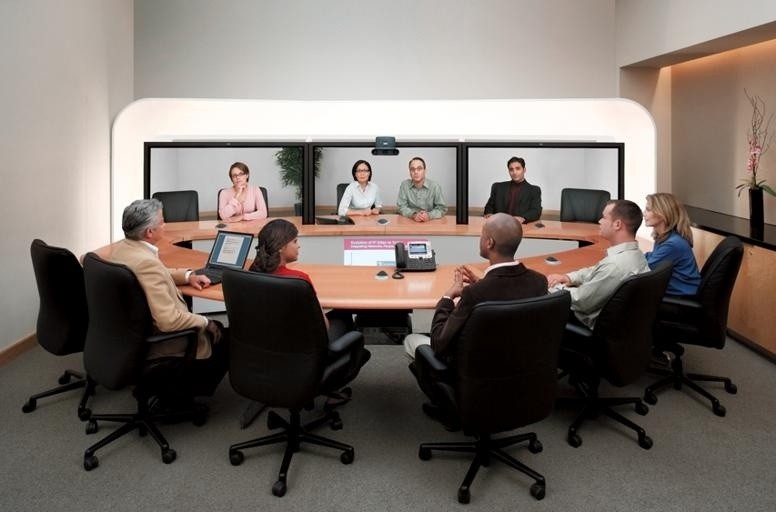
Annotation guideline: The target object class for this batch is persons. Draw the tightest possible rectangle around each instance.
[219,162,267,222]
[546,200,650,389]
[248,220,353,412]
[484,157,542,224]
[403,212,548,427]
[95,198,230,425]
[396,157,448,222]
[642,193,701,363]
[337,160,382,216]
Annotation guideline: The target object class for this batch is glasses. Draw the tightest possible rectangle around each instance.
[230,171,247,179]
[409,168,423,171]
[355,168,370,174]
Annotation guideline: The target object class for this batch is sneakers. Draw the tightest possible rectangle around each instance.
[422,401,449,426]
[322,387,353,412]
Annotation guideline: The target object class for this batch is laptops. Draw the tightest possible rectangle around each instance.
[193,228,254,287]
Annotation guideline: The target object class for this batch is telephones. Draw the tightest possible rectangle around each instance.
[395,241,436,272]
